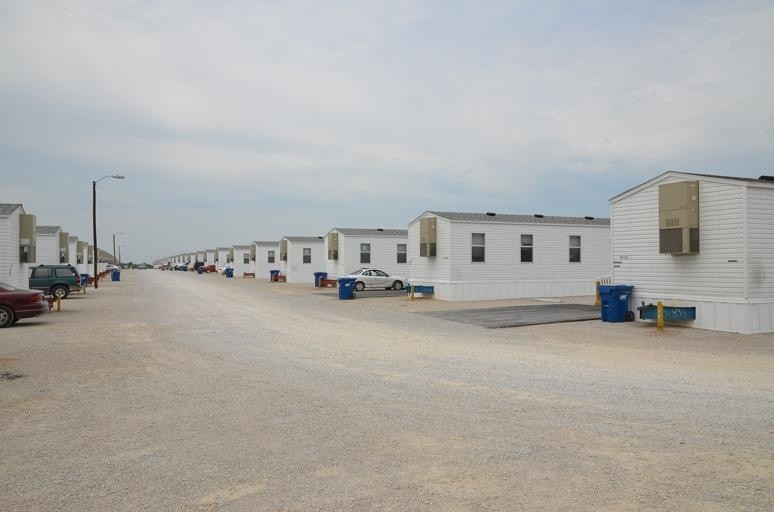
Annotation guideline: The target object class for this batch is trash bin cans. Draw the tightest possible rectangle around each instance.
[80,274,88,285]
[179,266,187,271]
[338,276,356,300]
[112,271,120,281]
[197,267,202,274]
[598,284,634,322]
[270,270,280,281]
[314,272,327,287]
[226,268,233,277]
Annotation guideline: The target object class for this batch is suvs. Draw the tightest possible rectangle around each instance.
[28,263,82,300]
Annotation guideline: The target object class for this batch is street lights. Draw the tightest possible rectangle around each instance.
[91,172,126,289]
[118,245,127,265]
[112,232,126,264]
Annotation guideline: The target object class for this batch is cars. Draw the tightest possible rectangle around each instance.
[160,262,233,274]
[0,281,49,329]
[336,267,408,291]
[138,264,147,269]
[105,264,121,272]
